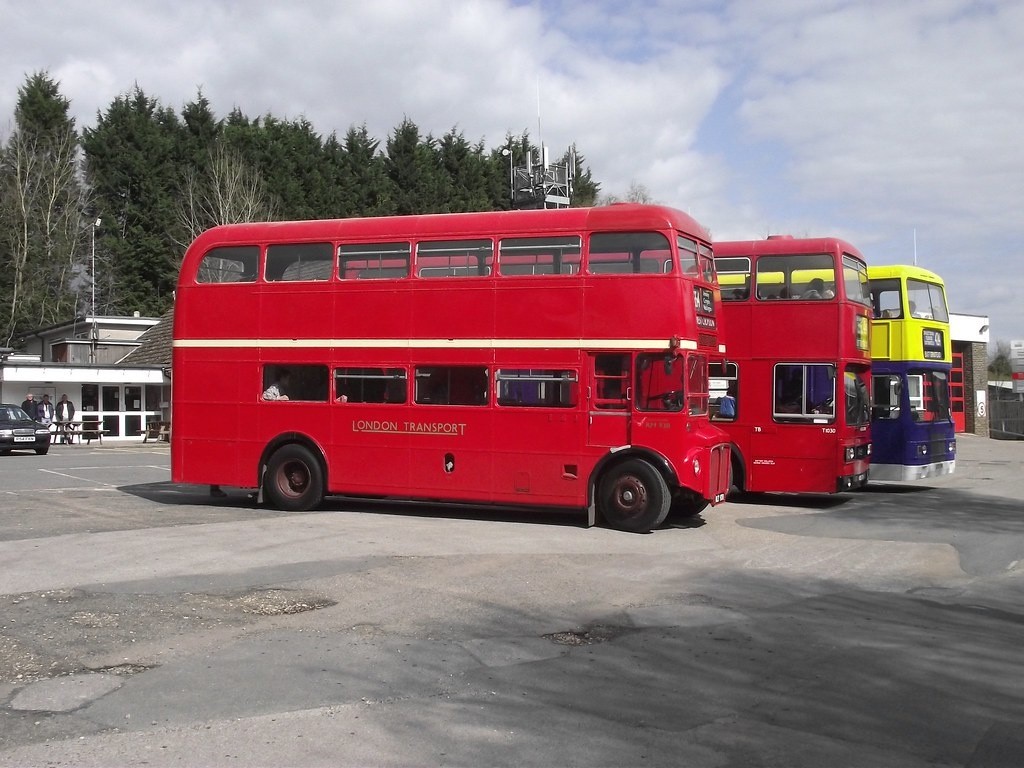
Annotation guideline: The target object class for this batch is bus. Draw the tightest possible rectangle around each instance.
[716,265,957,483]
[169,201,736,530]
[344,235,875,501]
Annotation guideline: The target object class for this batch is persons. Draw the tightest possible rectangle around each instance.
[37,394,54,423]
[22,393,38,420]
[210,484,228,497]
[262,368,291,401]
[55,394,75,443]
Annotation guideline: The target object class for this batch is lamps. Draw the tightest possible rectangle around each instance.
[979,324,989,334]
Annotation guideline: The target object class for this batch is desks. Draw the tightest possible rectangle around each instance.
[52,420,106,444]
[146,421,170,439]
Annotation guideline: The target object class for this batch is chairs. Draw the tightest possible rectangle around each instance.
[603,364,624,409]
[777,367,789,413]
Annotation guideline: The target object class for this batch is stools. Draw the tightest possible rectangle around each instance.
[136,430,170,443]
[50,430,111,445]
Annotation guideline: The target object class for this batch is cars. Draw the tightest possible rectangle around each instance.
[0,404,51,455]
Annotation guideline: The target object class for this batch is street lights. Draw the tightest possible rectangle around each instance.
[92,218,102,362]
[502,149,513,210]
